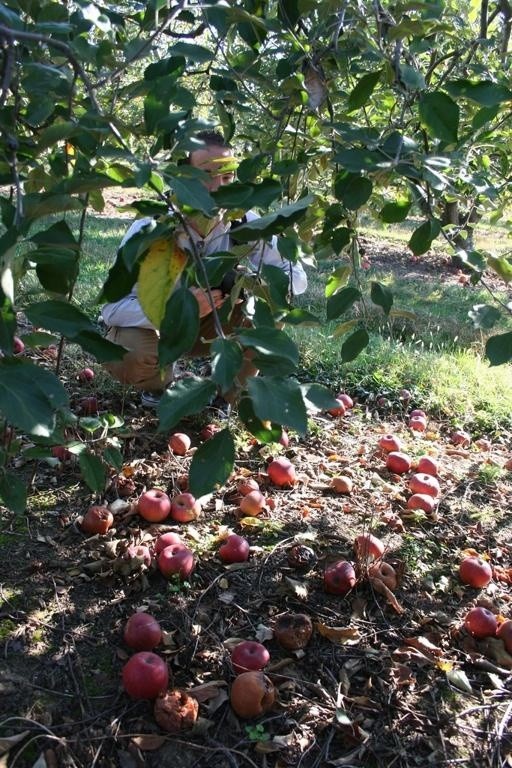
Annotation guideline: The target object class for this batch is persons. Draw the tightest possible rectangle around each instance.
[96,126,309,417]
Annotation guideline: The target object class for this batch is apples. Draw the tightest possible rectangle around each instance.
[10,338,512,733]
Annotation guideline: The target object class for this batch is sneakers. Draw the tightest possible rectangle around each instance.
[140,388,161,406]
